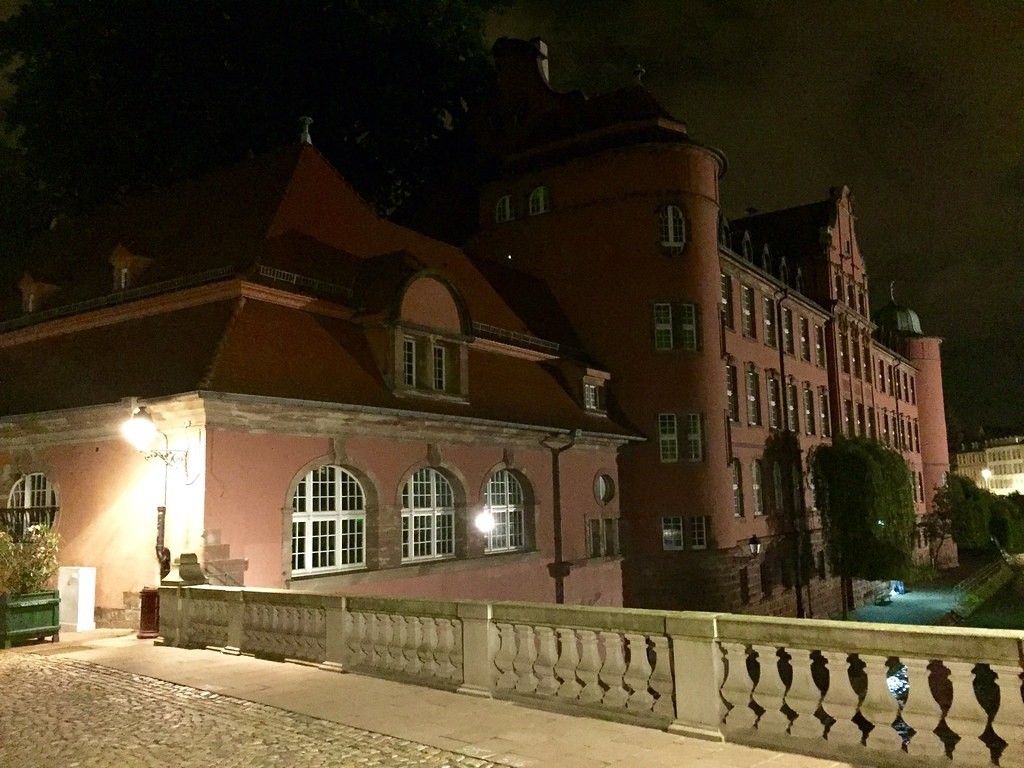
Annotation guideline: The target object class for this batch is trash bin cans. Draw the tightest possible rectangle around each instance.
[136,585,160,639]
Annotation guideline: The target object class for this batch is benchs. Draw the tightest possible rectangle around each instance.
[874,586,892,605]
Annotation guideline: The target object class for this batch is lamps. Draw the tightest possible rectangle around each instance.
[131,404,152,434]
[732,534,762,570]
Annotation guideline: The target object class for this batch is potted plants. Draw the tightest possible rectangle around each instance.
[0,517,61,648]
